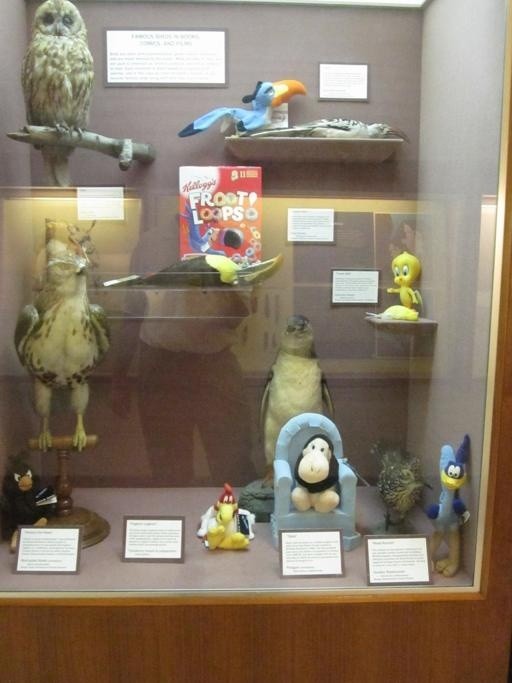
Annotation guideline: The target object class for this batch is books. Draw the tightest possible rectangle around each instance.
[176,164,264,264]
